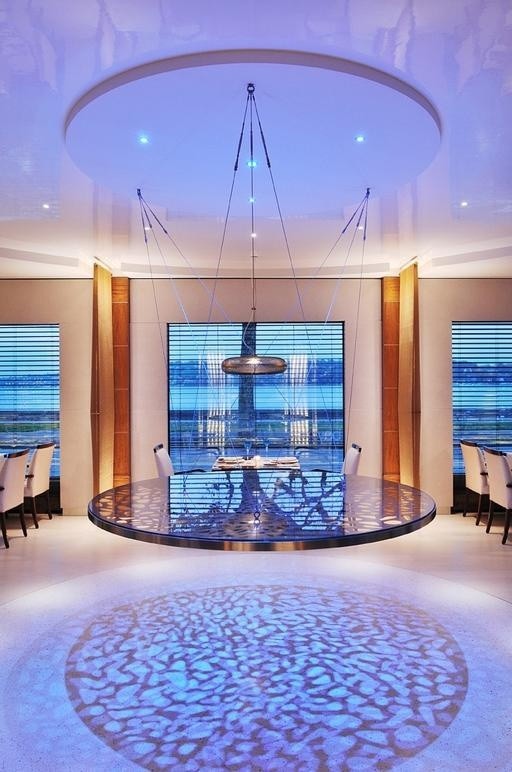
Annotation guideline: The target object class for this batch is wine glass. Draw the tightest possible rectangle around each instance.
[262,439,272,460]
[244,441,253,464]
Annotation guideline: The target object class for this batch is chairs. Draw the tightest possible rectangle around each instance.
[0,441,55,549]
[341,443,361,476]
[153,444,206,478]
[460,439,512,545]
